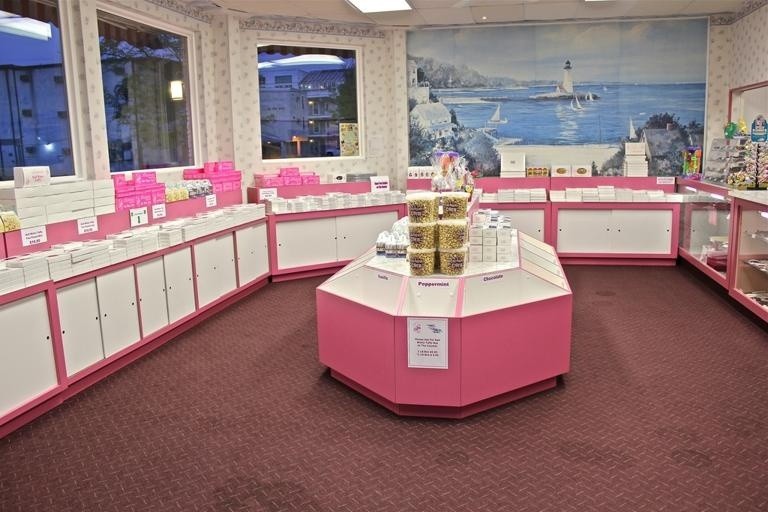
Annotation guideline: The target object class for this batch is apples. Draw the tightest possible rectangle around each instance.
[465,185,472,192]
[471,170,478,176]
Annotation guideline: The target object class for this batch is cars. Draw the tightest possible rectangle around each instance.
[326,145,340,156]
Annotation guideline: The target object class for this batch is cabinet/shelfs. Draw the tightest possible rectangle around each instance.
[701,136,750,187]
[553,202,678,262]
[312,218,575,426]
[56,265,141,378]
[477,207,548,248]
[237,222,269,287]
[136,246,196,337]
[1,289,60,424]
[194,232,237,309]
[680,178,768,319]
[270,207,404,286]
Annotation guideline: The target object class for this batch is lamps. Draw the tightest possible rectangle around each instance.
[161,58,186,103]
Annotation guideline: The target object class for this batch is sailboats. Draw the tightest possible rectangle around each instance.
[585,89,598,102]
[621,115,643,149]
[568,94,584,113]
[485,104,509,125]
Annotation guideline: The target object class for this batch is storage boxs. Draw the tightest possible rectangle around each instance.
[500,153,525,170]
